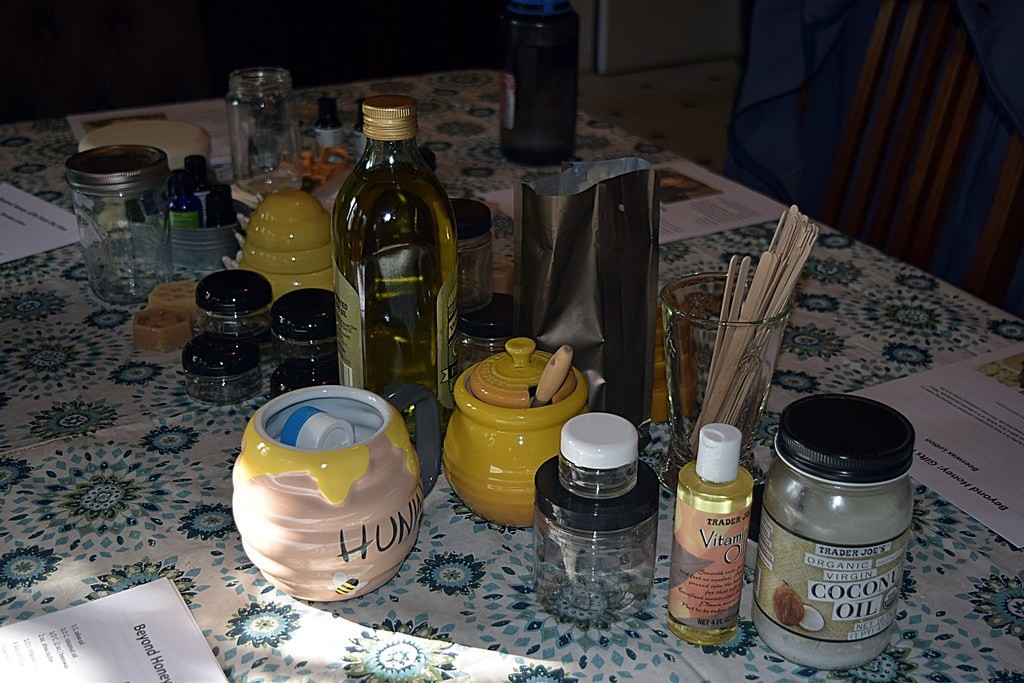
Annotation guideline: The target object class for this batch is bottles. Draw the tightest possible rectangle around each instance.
[227,67,304,195]
[497,0,582,166]
[532,457,661,628]
[668,422,753,646]
[450,292,514,376]
[313,97,345,157]
[751,395,915,672]
[332,95,457,450]
[442,338,588,528]
[168,156,232,227]
[449,199,495,316]
[354,98,366,160]
[64,143,172,305]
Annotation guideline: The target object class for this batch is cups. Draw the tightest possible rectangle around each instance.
[659,271,795,490]
[232,386,421,602]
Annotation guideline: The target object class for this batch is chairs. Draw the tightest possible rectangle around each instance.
[720,0,1024,327]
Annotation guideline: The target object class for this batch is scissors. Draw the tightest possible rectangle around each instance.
[281,147,349,194]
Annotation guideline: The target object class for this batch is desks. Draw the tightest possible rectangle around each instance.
[1,68,1024,683]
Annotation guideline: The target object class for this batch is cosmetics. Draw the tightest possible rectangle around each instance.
[668,423,754,646]
[532,412,658,623]
[168,95,513,407]
